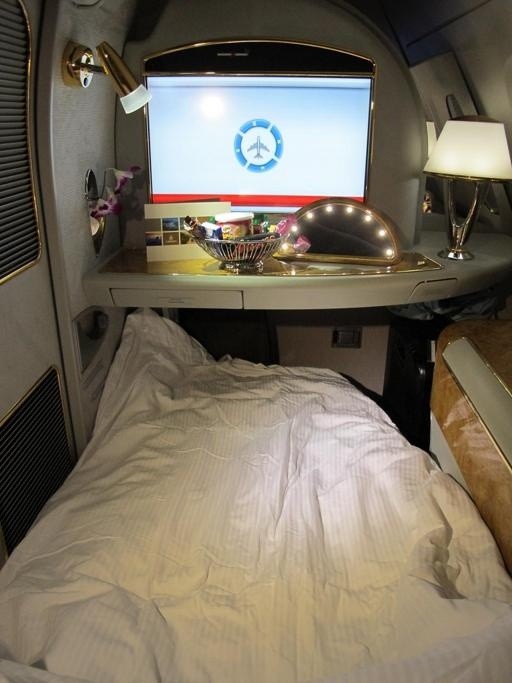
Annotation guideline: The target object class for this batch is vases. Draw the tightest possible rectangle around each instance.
[91,215,106,255]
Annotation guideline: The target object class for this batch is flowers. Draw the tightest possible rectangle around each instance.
[89,165,142,217]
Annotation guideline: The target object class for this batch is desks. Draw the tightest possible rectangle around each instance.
[81,230,512,310]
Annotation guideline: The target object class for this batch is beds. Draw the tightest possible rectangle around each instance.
[0,309,512,683]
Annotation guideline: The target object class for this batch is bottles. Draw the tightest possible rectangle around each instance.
[184,215,235,257]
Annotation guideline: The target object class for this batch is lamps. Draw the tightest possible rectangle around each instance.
[422,115,512,260]
[61,41,152,115]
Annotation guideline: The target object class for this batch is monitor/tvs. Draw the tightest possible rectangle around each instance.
[143,73,375,214]
